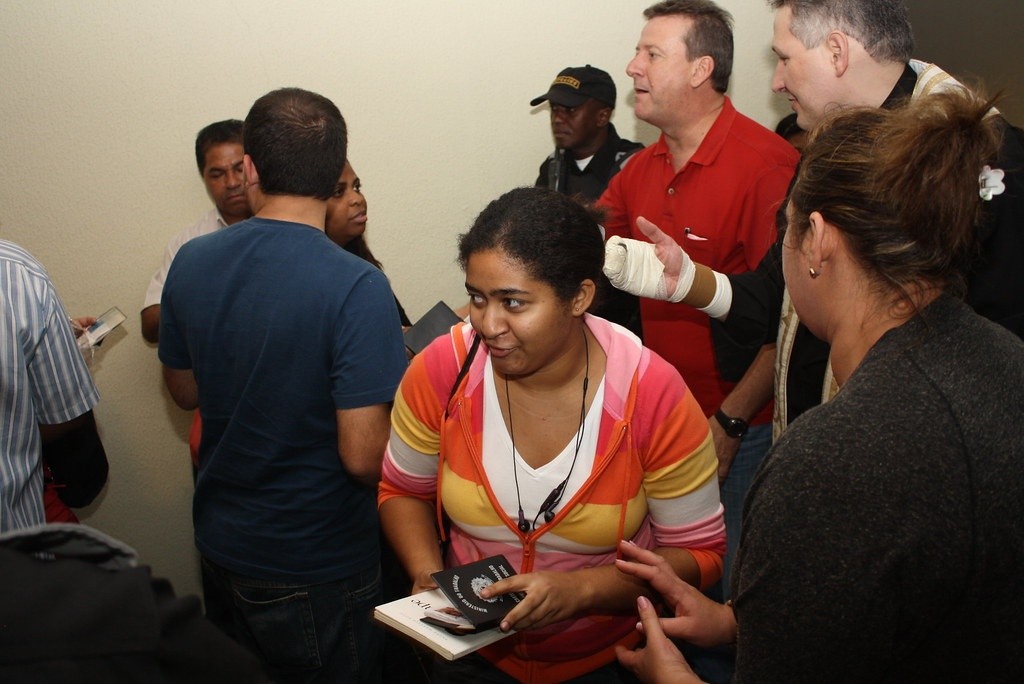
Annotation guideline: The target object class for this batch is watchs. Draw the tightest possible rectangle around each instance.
[715,409,748,438]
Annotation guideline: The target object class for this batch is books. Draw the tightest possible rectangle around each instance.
[404,301,464,353]
[373,587,518,661]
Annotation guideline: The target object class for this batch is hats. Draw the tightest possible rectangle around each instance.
[530,64,616,106]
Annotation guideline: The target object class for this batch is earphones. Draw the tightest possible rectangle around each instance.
[518,510,530,533]
[544,501,559,523]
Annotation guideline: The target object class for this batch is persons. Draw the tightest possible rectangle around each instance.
[0,239,269,684]
[141,119,255,344]
[156,88,409,684]
[530,65,645,203]
[191,159,471,487]
[378,188,728,683]
[774,114,807,149]
[603,0,1024,447]
[616,99,1024,684]
[584,0,802,604]
